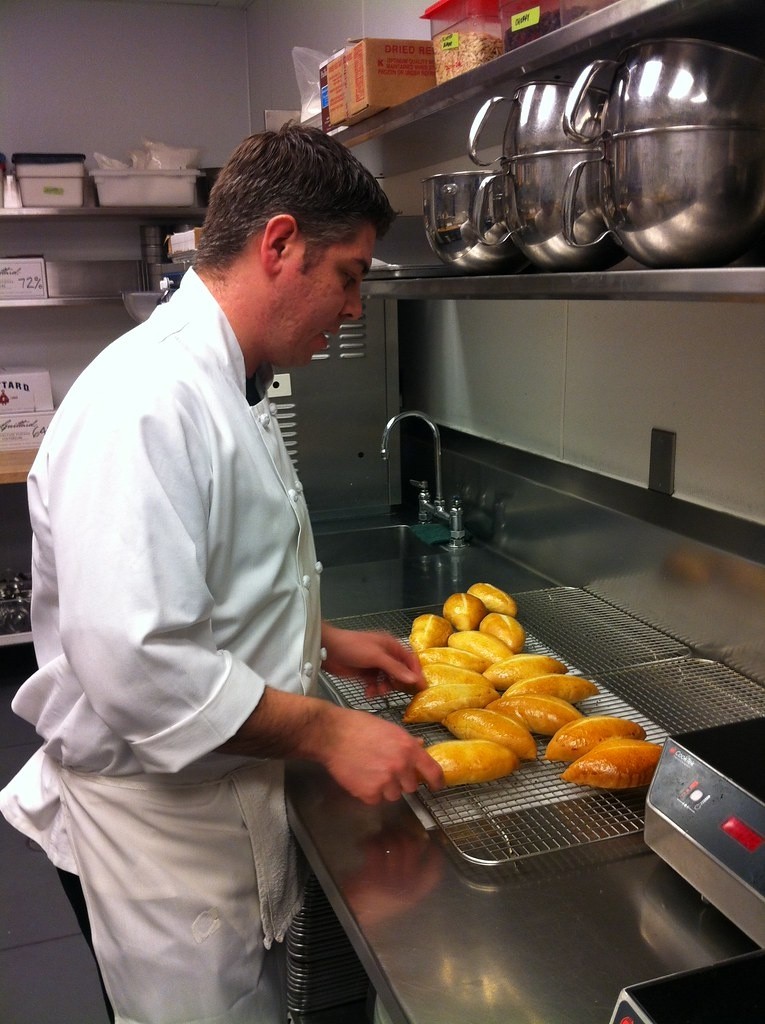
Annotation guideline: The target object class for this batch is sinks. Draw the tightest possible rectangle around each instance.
[313,524,451,570]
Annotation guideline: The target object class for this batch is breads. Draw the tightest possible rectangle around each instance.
[402,582,663,790]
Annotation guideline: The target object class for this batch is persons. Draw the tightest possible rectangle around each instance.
[1,119,444,1023]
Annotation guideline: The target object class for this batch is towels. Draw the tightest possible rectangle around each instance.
[228,758,311,951]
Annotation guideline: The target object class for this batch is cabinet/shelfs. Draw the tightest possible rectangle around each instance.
[0,207,211,309]
[333,0,765,299]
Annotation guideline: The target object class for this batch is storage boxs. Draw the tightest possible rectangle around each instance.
[0,137,208,452]
[318,0,619,132]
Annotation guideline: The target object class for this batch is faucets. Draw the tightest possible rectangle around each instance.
[379,410,469,552]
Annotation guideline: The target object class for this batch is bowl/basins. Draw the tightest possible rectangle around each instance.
[420,36,765,270]
